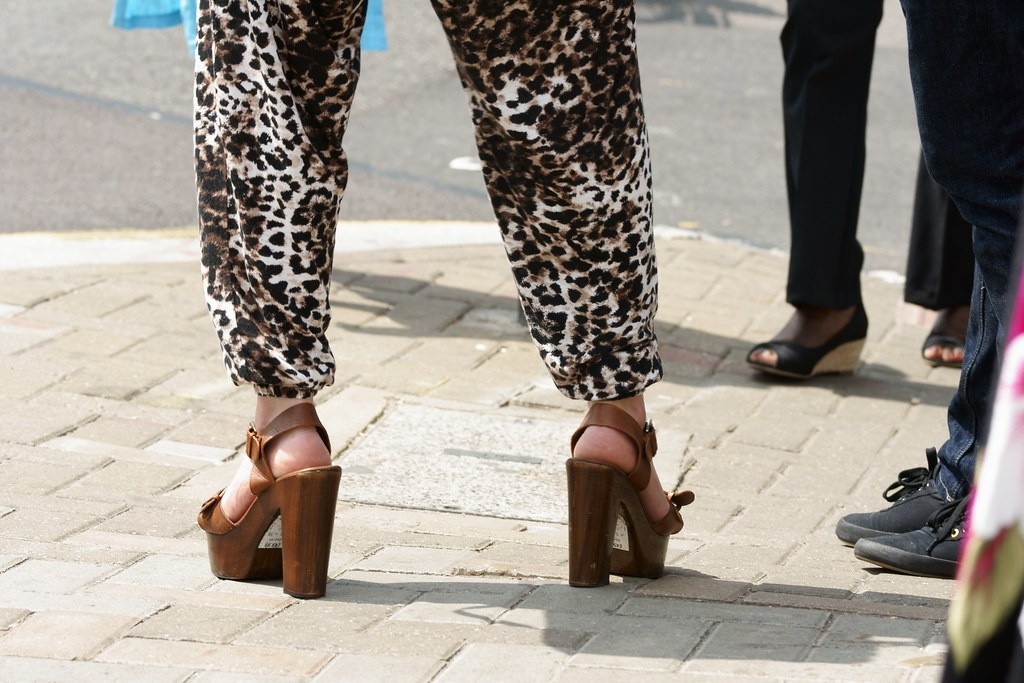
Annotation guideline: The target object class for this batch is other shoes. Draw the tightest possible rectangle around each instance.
[833,476,965,580]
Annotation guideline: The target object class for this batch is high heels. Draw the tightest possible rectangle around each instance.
[744,299,867,380]
[196,402,342,599]
[566,401,695,588]
[921,331,963,368]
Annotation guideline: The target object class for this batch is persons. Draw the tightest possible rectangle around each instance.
[197,1,684,599]
[746,0,977,379]
[837,0,1024,579]
